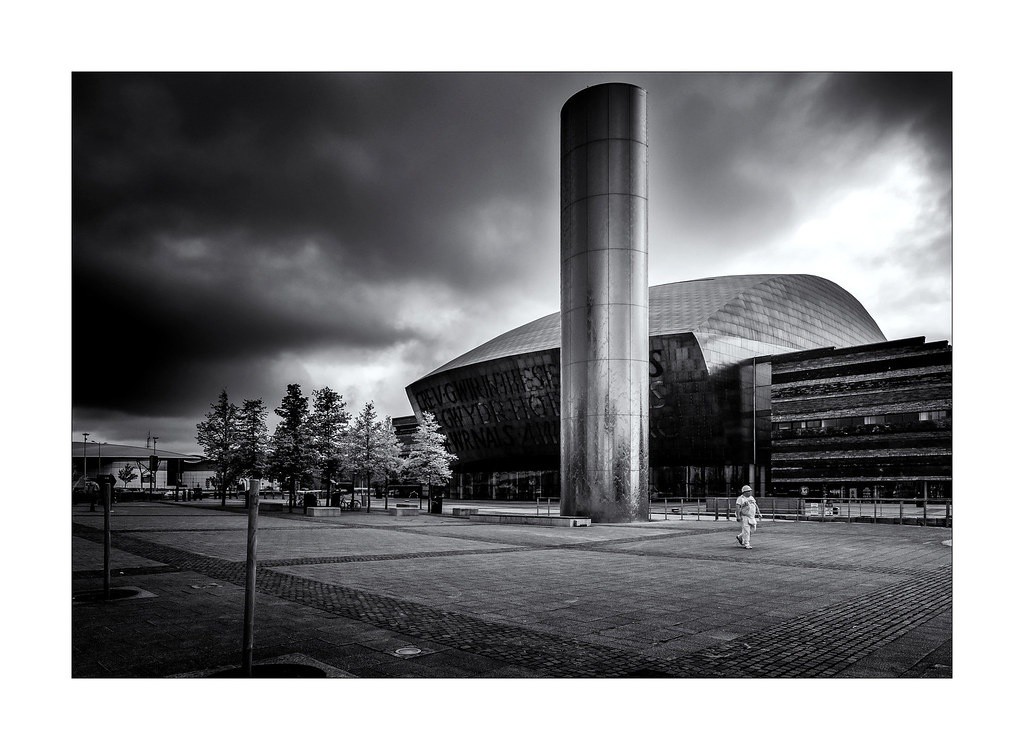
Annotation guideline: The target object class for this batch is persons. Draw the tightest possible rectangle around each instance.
[735,485,762,549]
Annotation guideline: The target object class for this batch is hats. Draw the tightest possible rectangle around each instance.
[742,485,753,493]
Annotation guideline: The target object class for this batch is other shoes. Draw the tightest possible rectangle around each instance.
[736,535,743,545]
[745,546,753,549]
